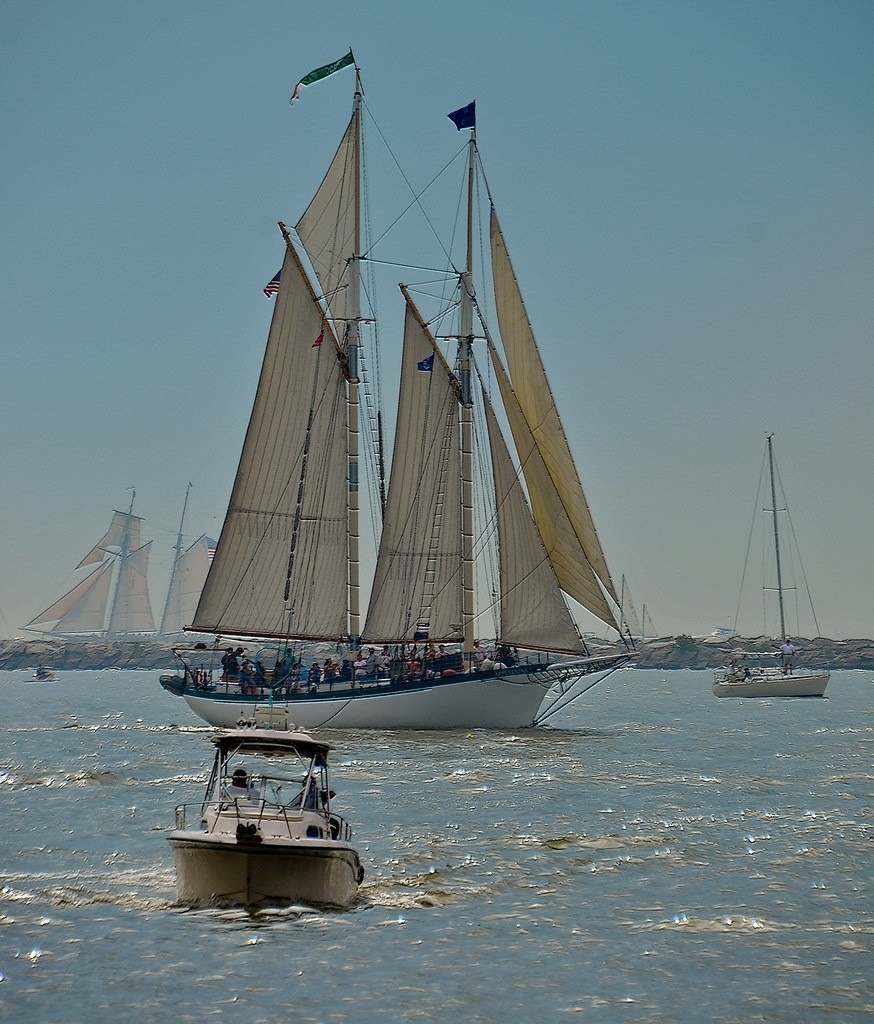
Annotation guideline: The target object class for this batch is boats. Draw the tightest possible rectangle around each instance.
[21,672,62,682]
[164,647,365,908]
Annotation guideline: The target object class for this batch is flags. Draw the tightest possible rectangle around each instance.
[205,537,217,561]
[447,99,476,131]
[311,328,324,348]
[417,354,434,372]
[262,269,280,298]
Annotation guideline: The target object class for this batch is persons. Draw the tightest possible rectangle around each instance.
[288,775,332,815]
[192,640,518,695]
[781,639,796,675]
[222,769,259,805]
[728,660,751,683]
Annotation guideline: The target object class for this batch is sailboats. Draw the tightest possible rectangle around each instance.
[713,432,831,698]
[17,481,215,642]
[157,46,641,732]
[599,573,660,641]
